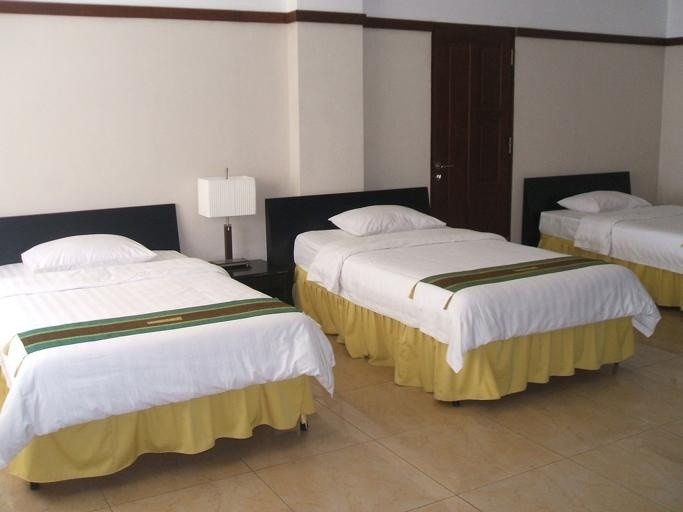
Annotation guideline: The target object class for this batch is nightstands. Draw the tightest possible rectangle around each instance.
[223,259,289,303]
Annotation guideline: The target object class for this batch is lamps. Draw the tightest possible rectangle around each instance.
[197,168,256,269]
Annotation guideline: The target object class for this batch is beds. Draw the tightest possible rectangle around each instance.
[265,186,662,406]
[521,172,683,311]
[0,204,336,490]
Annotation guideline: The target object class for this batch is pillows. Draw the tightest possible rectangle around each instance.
[20,234,157,275]
[557,190,652,214]
[327,205,447,236]
